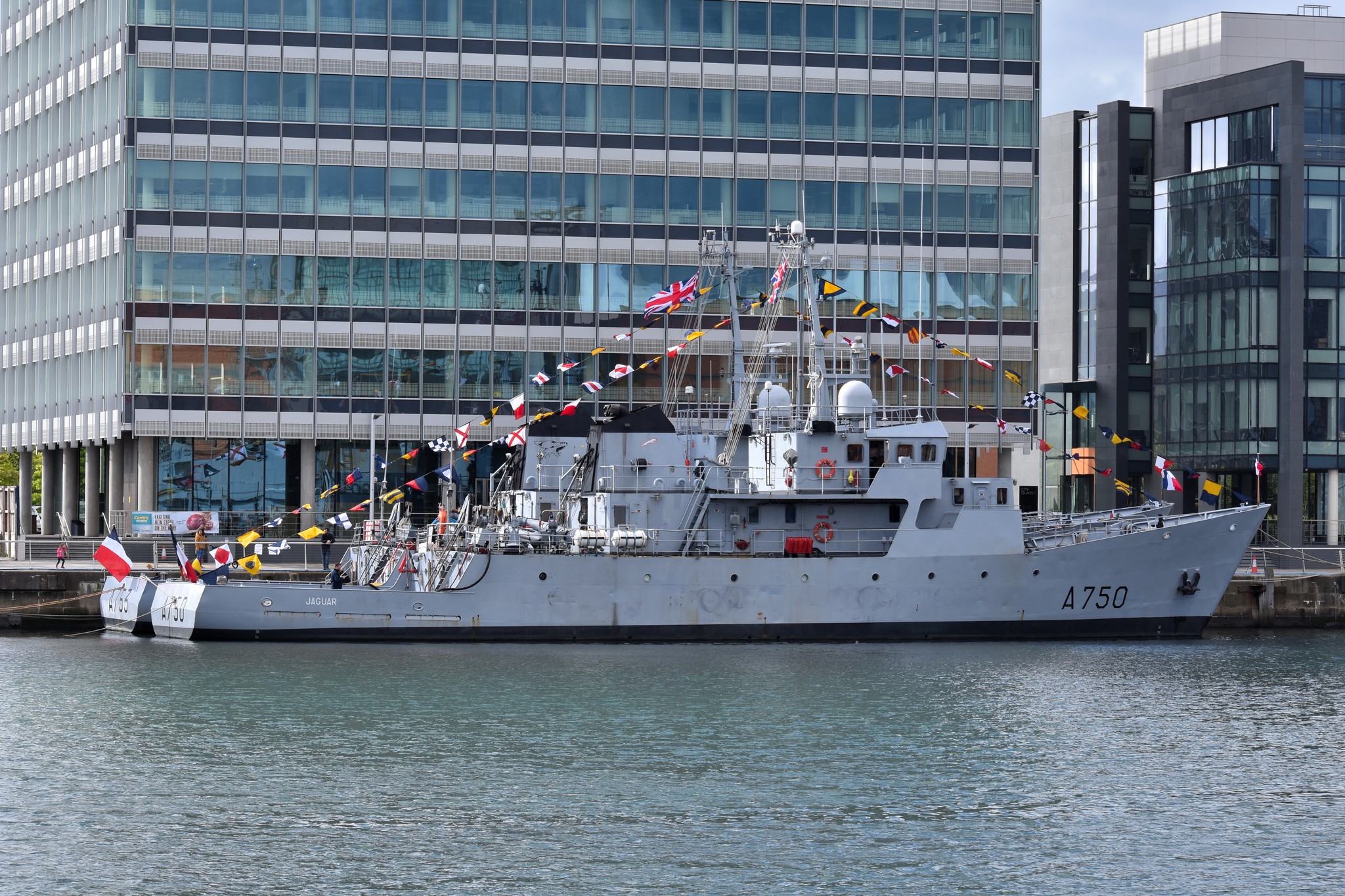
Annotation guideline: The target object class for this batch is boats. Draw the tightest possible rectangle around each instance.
[98,214,1274,646]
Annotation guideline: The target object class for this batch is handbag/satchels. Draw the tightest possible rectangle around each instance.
[195,547,197,554]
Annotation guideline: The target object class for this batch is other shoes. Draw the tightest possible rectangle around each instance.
[323,567,326,570]
[201,566,205,568]
[326,567,330,570]
[61,566,65,569]
[55,566,58,569]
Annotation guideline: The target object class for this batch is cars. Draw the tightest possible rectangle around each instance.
[31,506,42,532]
[79,501,85,522]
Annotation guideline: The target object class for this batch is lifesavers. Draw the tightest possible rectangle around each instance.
[374,535,390,545]
[815,458,835,480]
[812,521,834,543]
[232,561,238,569]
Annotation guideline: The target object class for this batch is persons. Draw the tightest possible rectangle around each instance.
[320,528,335,570]
[331,563,345,589]
[55,542,67,569]
[340,571,350,586]
[195,528,206,569]
[1083,503,1092,513]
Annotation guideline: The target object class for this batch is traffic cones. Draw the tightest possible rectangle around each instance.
[1250,555,1261,573]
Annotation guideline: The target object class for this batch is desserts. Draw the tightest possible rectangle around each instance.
[186,512,214,531]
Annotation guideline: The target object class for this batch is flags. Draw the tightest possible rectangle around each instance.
[1255,456,1264,476]
[173,251,788,583]
[92,526,134,583]
[818,274,1256,506]
